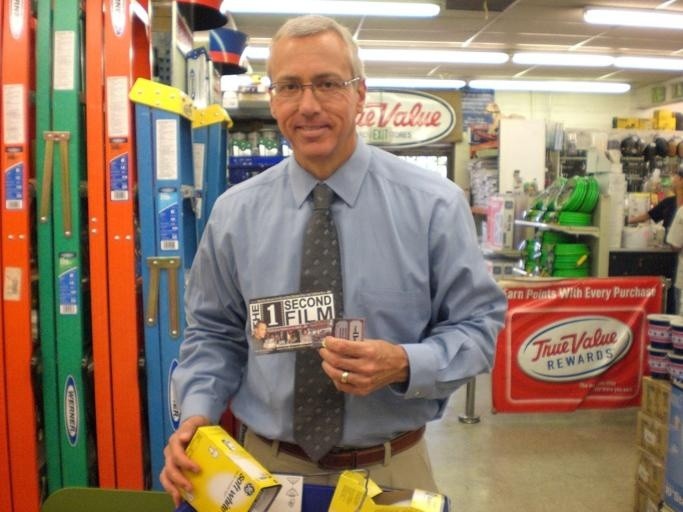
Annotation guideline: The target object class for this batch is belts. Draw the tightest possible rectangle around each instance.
[254,424,426,471]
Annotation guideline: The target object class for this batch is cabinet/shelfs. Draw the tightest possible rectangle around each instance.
[510,219,599,281]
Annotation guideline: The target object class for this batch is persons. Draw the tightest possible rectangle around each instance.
[664,184,683,319]
[157,12,509,507]
[627,170,683,317]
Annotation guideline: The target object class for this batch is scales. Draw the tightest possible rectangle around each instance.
[483,250,521,282]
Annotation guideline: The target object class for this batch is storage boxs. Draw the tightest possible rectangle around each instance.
[629,374,683,512]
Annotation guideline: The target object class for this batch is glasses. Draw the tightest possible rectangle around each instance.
[267,77,360,97]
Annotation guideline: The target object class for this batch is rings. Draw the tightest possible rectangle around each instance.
[339,370,348,384]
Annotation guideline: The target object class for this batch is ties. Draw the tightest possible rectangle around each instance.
[292,184,344,466]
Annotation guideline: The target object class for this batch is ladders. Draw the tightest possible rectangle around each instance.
[35,0,98,490]
[187,48,230,234]
[0,0,41,508]
[132,84,194,490]
[84,0,154,493]
